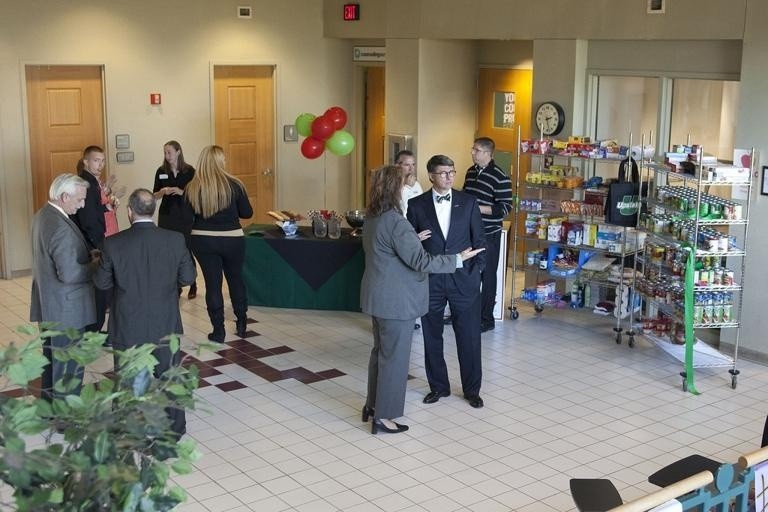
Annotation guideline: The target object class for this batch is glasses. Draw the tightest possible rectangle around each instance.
[432,170,456,178]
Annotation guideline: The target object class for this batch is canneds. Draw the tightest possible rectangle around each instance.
[644,233,733,286]
[656,185,742,222]
[635,268,731,324]
[638,212,734,252]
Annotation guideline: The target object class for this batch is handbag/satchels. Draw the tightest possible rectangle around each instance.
[605,179,648,226]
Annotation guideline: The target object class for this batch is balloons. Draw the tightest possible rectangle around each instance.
[295,107,355,160]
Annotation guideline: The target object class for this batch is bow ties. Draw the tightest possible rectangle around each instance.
[436,193,450,203]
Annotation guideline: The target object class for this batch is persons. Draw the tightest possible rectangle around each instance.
[445,137,513,333]
[77,159,119,238]
[28,173,100,435]
[361,166,486,434]
[407,155,486,408]
[180,145,254,343]
[74,146,110,334]
[395,151,424,217]
[93,188,197,446]
[153,141,200,300]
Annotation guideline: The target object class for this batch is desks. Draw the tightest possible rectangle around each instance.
[242,223,371,313]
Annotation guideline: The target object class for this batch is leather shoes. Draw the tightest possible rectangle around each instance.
[464,393,483,408]
[423,390,450,403]
[208,328,225,342]
[481,321,494,333]
[188,287,197,299]
[236,319,246,333]
[444,317,452,324]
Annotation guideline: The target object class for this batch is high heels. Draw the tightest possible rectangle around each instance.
[362,405,408,434]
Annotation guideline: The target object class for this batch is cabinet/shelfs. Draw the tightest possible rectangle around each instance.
[512,122,655,343]
[640,133,755,393]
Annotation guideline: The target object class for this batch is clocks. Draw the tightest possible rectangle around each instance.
[534,102,565,135]
